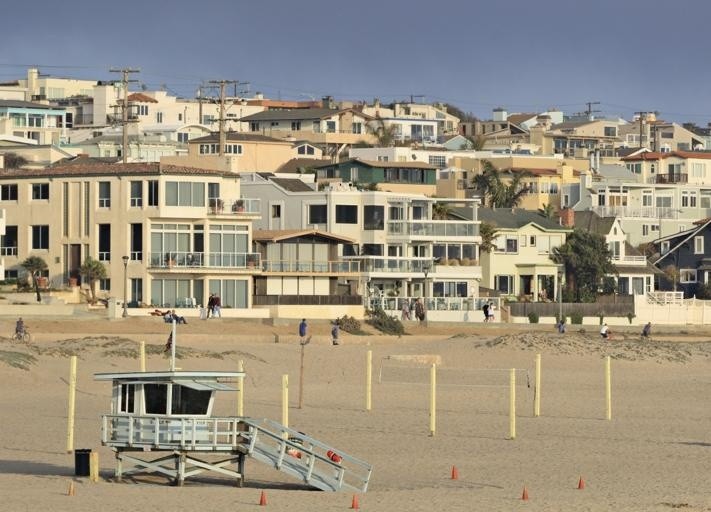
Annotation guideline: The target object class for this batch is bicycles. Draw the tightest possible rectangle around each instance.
[11,326,30,343]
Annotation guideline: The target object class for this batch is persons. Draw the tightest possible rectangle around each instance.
[402,298,423,321]
[558,320,565,333]
[600,324,613,339]
[331,322,340,345]
[540,288,548,303]
[643,322,652,340]
[16,318,24,340]
[207,292,222,319]
[483,301,496,323]
[164,310,188,324]
[299,319,308,344]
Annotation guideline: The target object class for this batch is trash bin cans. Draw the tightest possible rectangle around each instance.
[107,296,124,319]
[75,449,91,477]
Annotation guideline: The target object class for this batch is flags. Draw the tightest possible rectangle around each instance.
[164,331,172,352]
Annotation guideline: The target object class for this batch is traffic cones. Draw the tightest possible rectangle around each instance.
[522,484,529,500]
[576,476,586,489]
[350,493,360,509]
[451,466,457,479]
[258,489,268,506]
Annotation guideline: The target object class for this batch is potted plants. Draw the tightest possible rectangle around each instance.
[236,195,245,214]
[210,198,224,214]
[246,253,257,271]
[164,250,178,269]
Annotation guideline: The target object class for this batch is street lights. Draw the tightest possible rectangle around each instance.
[556,271,563,322]
[122,255,130,318]
[422,266,428,319]
